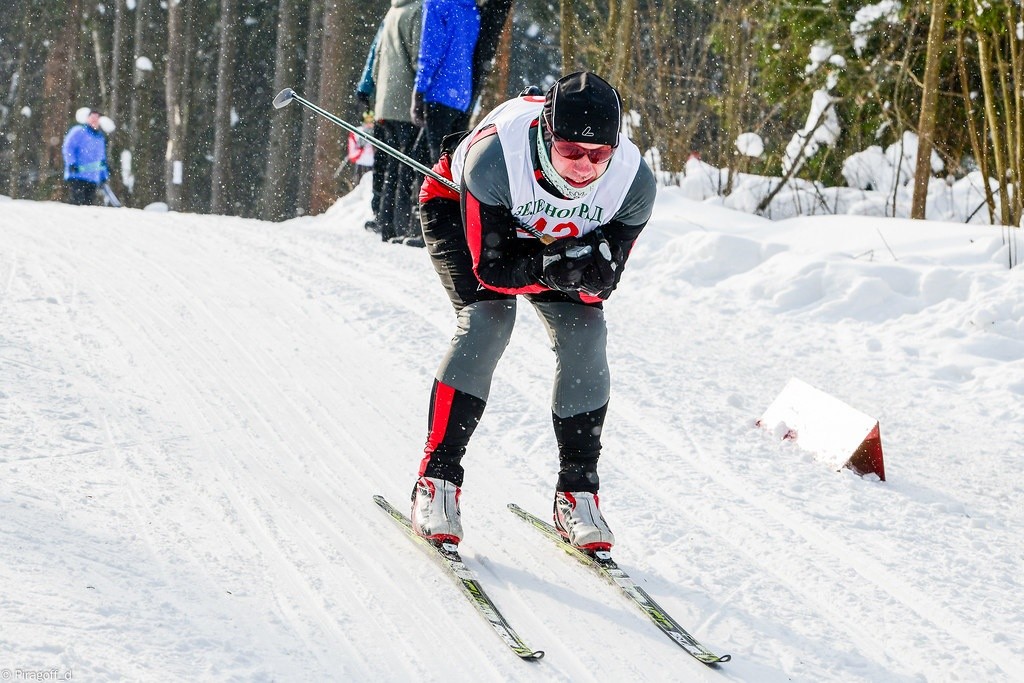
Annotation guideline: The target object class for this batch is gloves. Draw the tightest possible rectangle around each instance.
[69,163,79,171]
[540,230,618,295]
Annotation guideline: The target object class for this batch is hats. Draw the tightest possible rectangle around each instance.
[542,71,623,148]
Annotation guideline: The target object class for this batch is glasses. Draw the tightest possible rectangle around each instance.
[547,126,617,164]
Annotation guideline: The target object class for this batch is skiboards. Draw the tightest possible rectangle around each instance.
[370,494,732,666]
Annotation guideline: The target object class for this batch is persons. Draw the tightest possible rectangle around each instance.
[62,111,109,206]
[355,0,513,248]
[412,69,656,552]
[347,112,374,182]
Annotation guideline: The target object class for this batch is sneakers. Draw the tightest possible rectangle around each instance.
[411,477,463,543]
[553,489,615,550]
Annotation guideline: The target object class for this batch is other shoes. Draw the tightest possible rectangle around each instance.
[365,217,425,247]
